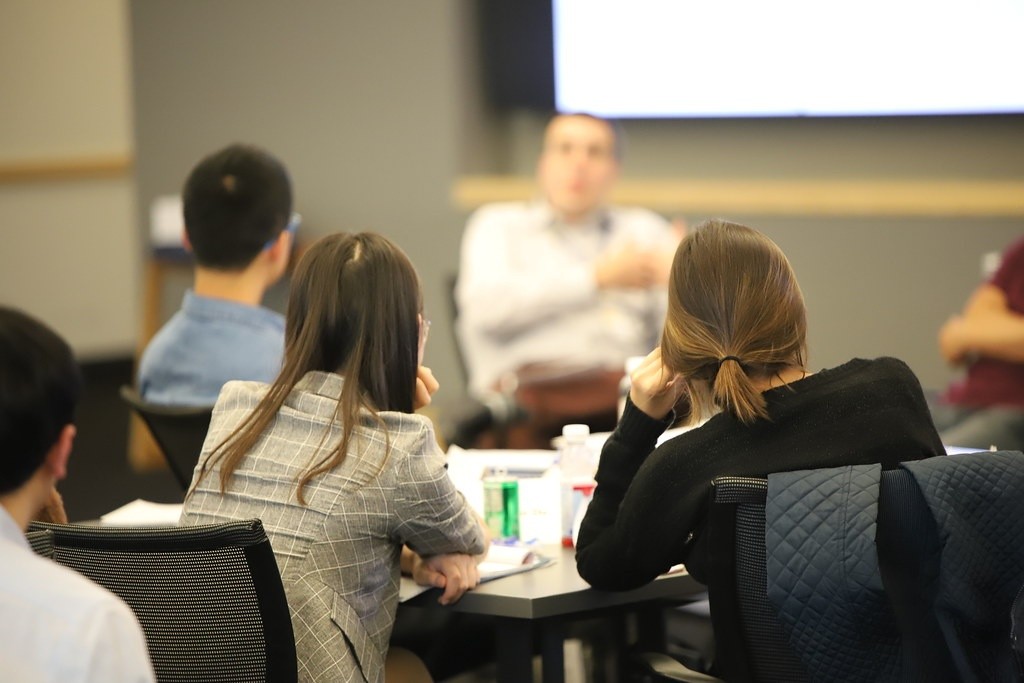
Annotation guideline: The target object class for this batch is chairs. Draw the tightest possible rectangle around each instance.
[714,448,1023,682]
[113,386,220,495]
[27,519,299,683]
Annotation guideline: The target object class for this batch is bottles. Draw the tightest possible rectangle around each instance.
[557,420,593,549]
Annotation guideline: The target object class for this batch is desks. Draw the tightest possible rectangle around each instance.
[392,447,709,683]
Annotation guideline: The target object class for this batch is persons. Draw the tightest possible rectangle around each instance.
[451,114,683,418]
[178,232,492,682]
[138,141,300,406]
[575,218,948,682]
[930,229,1024,452]
[0,304,157,683]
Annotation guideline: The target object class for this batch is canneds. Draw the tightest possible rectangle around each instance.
[481,474,520,547]
